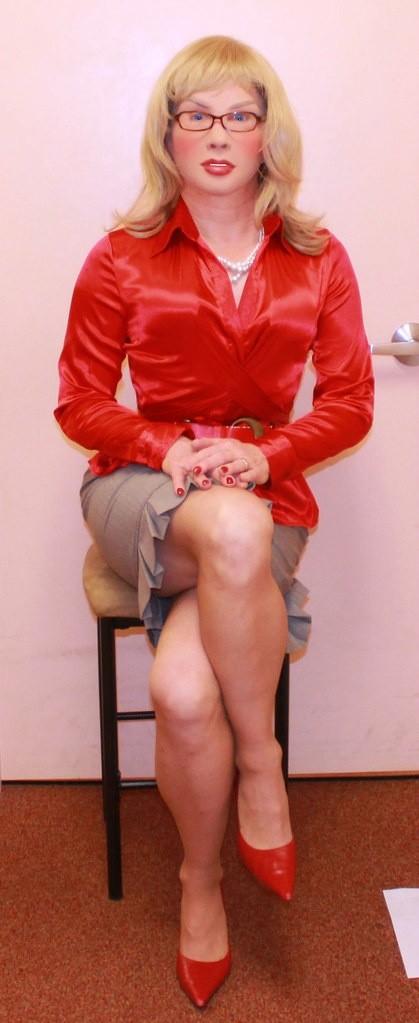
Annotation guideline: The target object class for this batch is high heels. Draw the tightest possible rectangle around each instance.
[178,885,232,1010]
[236,807,293,902]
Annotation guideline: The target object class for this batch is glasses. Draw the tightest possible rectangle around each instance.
[171,110,265,133]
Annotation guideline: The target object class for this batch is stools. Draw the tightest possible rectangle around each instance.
[77,530,297,912]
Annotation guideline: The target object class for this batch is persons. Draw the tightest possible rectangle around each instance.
[49,32,377,1012]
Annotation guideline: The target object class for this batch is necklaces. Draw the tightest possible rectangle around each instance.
[214,214,267,285]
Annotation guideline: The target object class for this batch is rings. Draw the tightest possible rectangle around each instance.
[238,458,251,472]
